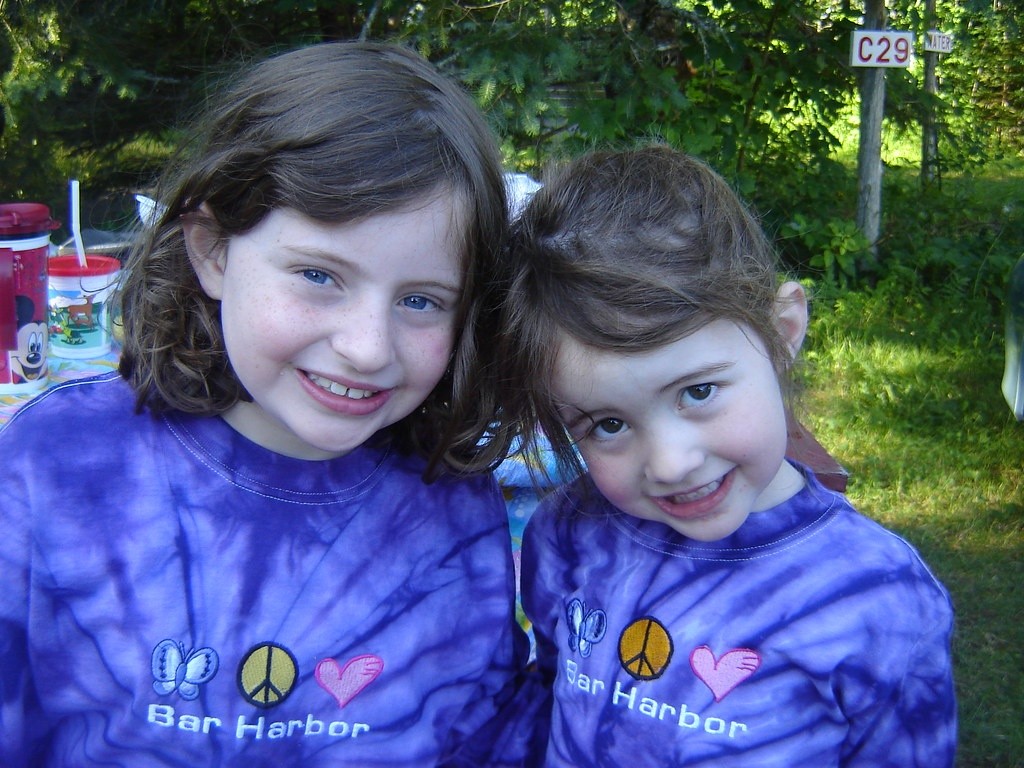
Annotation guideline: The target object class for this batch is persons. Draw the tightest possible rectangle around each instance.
[1,39,516,768]
[497,142,959,768]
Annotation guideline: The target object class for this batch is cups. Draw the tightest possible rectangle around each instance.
[47,254,120,358]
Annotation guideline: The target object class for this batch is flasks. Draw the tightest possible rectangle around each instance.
[0,203,63,395]
